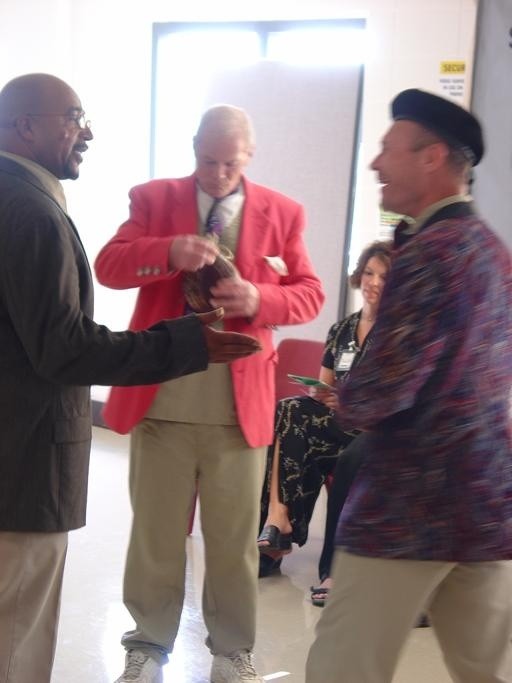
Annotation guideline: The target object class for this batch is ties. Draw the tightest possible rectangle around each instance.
[184,177,238,316]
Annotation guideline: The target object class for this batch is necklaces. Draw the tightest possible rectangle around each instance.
[359,310,380,324]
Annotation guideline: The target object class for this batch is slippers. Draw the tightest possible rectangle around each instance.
[257,525,291,550]
[259,553,282,577]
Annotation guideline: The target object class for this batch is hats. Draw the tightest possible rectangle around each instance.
[392,89,483,166]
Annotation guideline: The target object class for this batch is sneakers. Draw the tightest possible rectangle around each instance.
[210,653,264,683]
[114,651,162,683]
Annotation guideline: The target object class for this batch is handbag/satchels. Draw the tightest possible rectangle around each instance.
[167,232,241,314]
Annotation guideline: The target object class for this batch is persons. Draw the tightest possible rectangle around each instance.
[90,102,327,683]
[302,85,512,683]
[307,431,367,607]
[241,242,401,582]
[1,71,266,683]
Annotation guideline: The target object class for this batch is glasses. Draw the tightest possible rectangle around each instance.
[24,112,91,131]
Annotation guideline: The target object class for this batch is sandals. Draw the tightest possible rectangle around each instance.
[310,573,331,606]
